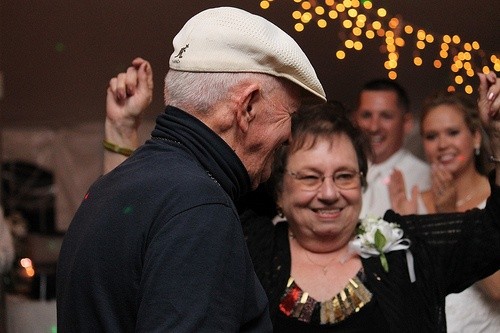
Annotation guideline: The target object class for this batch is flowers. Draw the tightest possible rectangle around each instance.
[349,215,415,278]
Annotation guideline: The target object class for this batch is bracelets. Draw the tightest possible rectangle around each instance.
[488,155,500,163]
[103,141,134,156]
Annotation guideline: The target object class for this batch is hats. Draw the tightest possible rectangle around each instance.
[168,7,326,106]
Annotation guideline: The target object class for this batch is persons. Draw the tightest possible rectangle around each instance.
[348,79,435,217]
[102,58,500,333]
[390,97,500,333]
[54,5,327,333]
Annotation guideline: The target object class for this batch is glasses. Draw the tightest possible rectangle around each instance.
[281,167,367,193]
